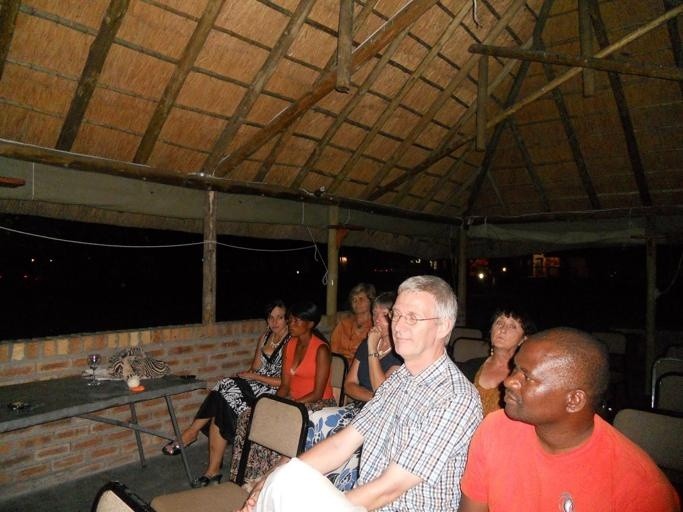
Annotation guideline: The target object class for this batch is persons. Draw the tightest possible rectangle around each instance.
[233,274,484,512]
[331,283,376,405]
[230,301,337,486]
[461,303,538,420]
[161,298,292,488]
[457,326,681,512]
[305,291,404,492]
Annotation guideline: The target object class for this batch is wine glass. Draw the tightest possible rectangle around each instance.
[86,354,101,386]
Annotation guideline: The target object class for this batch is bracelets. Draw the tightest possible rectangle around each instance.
[368,352,379,357]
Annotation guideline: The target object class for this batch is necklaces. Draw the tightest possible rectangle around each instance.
[270,331,288,349]
[356,322,363,329]
[376,338,392,356]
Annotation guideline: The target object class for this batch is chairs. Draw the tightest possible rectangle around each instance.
[452,337,490,362]
[613,408,683,497]
[330,352,348,405]
[148,394,308,511]
[90,481,152,512]
[652,357,683,415]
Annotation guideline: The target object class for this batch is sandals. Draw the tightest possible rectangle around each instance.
[162,437,198,456]
[191,473,223,487]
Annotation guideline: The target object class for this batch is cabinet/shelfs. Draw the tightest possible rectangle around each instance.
[449,326,482,345]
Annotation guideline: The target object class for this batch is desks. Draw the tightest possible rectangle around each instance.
[0,370,207,488]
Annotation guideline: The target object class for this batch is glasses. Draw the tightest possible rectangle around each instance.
[387,309,442,326]
